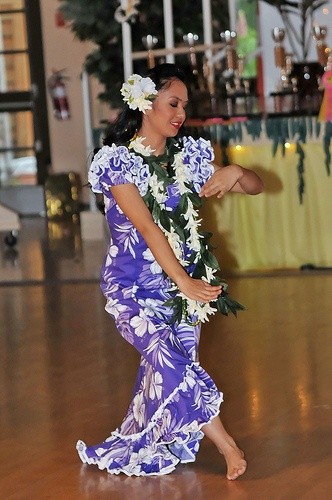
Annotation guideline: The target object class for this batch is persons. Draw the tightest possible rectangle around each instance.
[75,63,264,481]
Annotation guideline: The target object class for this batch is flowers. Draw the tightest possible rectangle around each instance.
[120,74,158,115]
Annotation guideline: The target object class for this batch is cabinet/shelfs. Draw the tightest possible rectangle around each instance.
[0,0,51,218]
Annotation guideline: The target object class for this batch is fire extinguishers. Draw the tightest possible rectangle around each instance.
[48,69,71,120]
